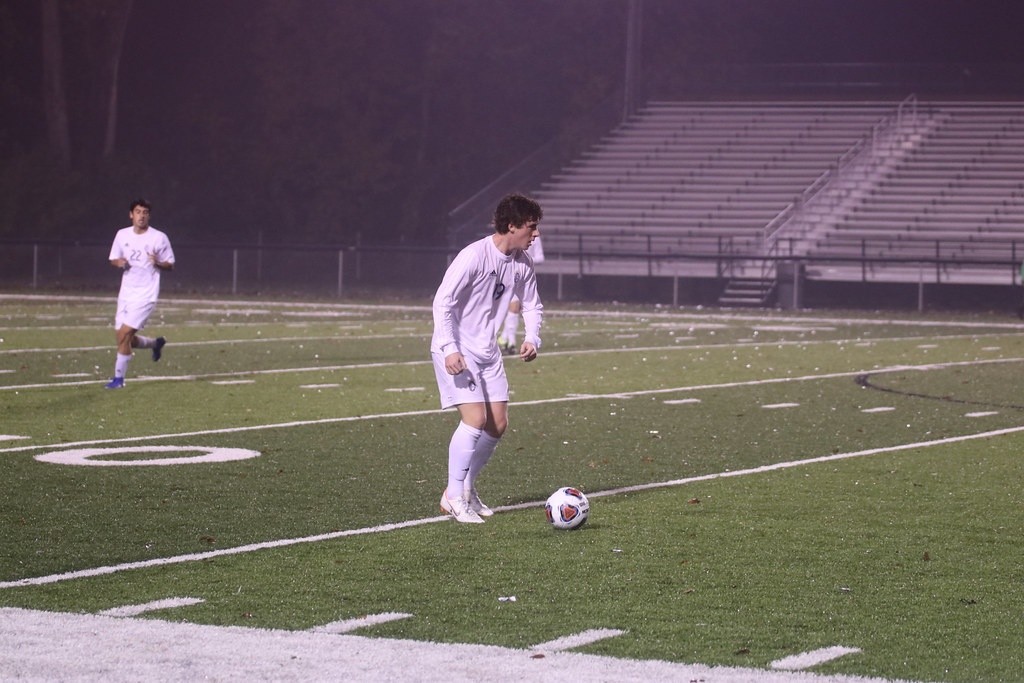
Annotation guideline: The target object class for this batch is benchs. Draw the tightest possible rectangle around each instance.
[462,99,1024,276]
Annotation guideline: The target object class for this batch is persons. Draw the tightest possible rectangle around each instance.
[428,191,546,524]
[104,195,175,390]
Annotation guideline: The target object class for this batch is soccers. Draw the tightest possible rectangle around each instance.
[546,486,590,531]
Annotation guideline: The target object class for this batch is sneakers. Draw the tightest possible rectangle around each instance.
[105,378,125,390]
[464,487,493,518]
[440,490,485,525]
[153,336,165,362]
